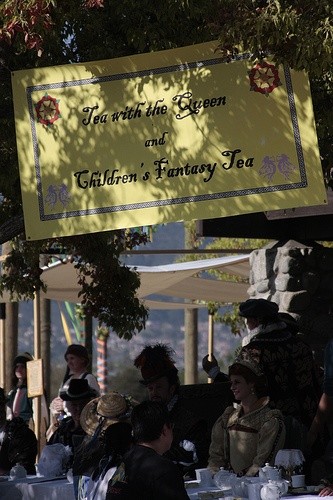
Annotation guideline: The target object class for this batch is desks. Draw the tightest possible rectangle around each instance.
[0,474,78,500]
[185,480,333,500]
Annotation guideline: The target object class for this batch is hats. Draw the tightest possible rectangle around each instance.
[0,387,9,406]
[80,393,141,438]
[237,299,280,320]
[13,352,34,365]
[59,379,99,402]
[65,344,88,363]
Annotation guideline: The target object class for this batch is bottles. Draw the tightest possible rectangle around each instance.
[67,468,73,483]
[10,463,27,480]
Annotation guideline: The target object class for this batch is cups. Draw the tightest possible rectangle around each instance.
[291,475,305,488]
[195,468,212,487]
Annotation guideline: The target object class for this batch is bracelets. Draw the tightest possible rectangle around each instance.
[208,366,220,379]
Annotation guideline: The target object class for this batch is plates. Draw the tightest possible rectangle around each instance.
[288,488,322,495]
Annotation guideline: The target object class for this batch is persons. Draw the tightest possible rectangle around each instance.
[70,391,189,500]
[0,351,49,475]
[207,361,287,477]
[47,378,99,448]
[237,298,333,498]
[129,343,235,473]
[57,344,100,396]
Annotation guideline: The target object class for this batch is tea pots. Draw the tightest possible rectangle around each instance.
[214,463,289,500]
[34,457,58,476]
